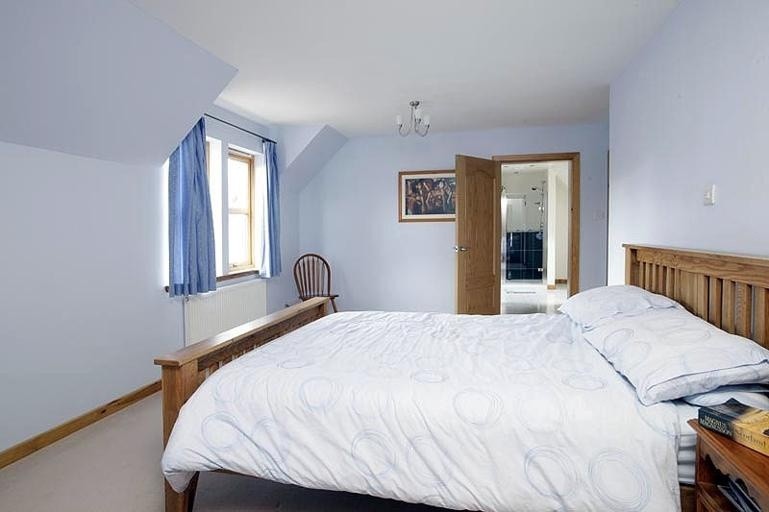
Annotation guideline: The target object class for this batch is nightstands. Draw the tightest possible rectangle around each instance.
[688,405,769,511]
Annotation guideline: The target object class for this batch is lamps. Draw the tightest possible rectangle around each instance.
[396,99,431,137]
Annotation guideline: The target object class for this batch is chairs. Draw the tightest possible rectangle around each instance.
[292,251,341,313]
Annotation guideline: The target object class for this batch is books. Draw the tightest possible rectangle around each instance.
[698,398,769,457]
[716,479,763,512]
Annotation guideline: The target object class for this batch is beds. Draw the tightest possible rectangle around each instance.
[152,239,768,511]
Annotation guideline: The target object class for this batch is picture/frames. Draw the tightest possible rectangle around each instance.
[397,169,457,222]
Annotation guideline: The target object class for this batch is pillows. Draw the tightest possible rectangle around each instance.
[577,309,766,405]
[556,283,679,329]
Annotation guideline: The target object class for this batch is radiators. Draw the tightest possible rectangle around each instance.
[184,278,266,348]
